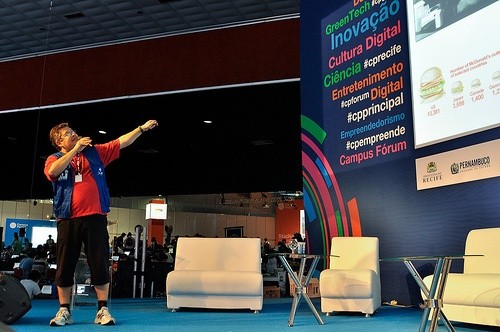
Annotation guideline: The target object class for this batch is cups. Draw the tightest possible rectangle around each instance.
[297,242,306,255]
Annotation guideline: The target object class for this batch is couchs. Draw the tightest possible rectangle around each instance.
[166,237,263,314]
[319,237,381,318]
[421,228,500,326]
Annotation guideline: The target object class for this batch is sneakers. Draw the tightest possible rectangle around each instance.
[94,306,116,325]
[49,306,73,326]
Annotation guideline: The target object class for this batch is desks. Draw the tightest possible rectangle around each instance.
[378,255,483,332]
[266,253,340,327]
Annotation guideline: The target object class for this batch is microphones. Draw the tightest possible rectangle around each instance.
[78,136,93,147]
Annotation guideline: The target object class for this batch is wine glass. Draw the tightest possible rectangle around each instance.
[288,239,298,254]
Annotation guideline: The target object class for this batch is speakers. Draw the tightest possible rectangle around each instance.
[0,274,32,325]
[111,272,141,298]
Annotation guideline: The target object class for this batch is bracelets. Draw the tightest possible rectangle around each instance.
[138,126,144,134]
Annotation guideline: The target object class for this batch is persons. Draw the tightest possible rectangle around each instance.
[110,232,168,297]
[0,232,57,300]
[257,231,307,276]
[44,119,159,326]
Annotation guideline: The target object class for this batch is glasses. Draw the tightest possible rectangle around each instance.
[61,130,76,139]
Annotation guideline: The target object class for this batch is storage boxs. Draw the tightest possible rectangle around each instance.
[287,270,320,298]
